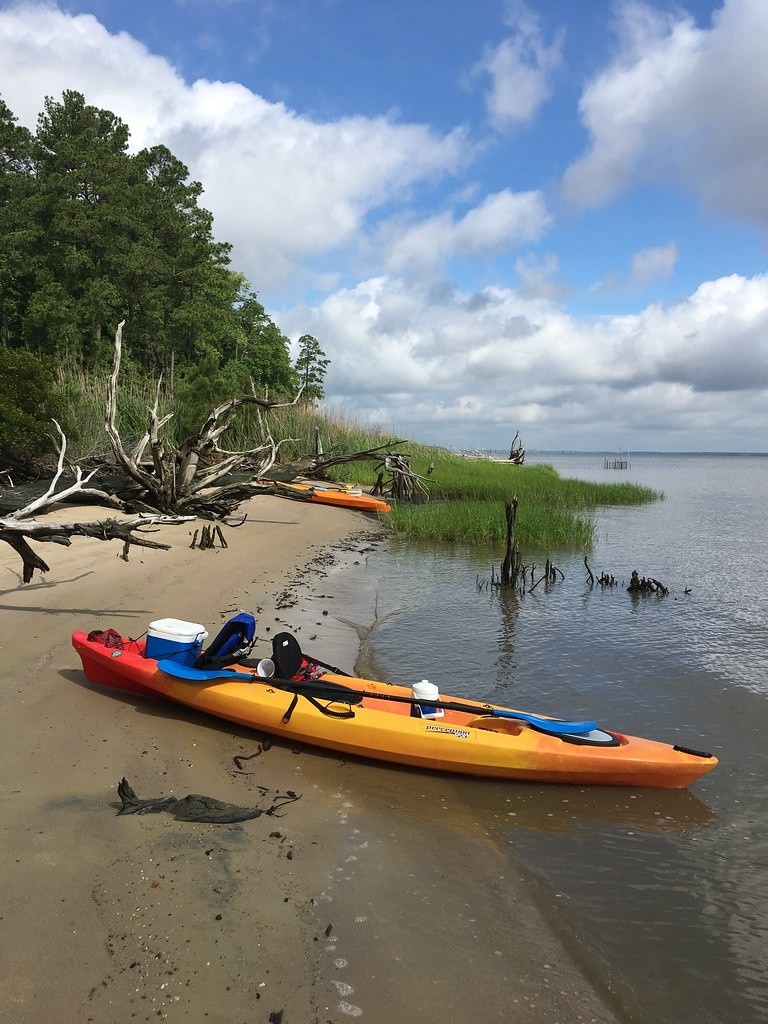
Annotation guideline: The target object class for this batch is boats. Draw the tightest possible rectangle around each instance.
[260,481,391,513]
[72,630,719,791]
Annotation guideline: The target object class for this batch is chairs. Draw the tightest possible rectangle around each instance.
[272,632,302,680]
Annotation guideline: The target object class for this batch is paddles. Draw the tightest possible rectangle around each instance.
[156,657,598,736]
[335,487,363,496]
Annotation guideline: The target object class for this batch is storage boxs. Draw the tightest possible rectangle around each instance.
[145,618,209,668]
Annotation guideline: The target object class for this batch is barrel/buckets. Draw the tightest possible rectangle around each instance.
[410,680,444,721]
[256,659,275,678]
